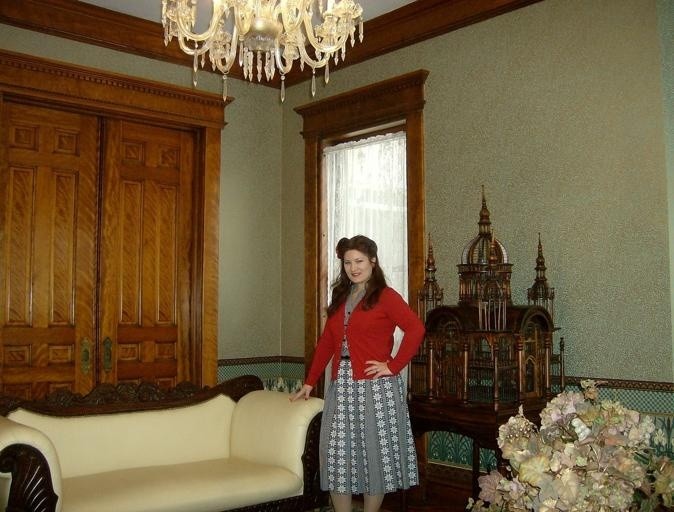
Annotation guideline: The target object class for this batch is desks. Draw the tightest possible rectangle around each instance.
[410,397,553,502]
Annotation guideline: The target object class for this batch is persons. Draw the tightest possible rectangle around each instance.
[288,235,426,512]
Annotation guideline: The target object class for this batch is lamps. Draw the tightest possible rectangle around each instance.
[161,0,364,103]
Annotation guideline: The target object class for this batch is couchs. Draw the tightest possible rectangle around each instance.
[0,374,325,512]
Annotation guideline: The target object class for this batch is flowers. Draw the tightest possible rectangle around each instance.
[466,379,674,512]
